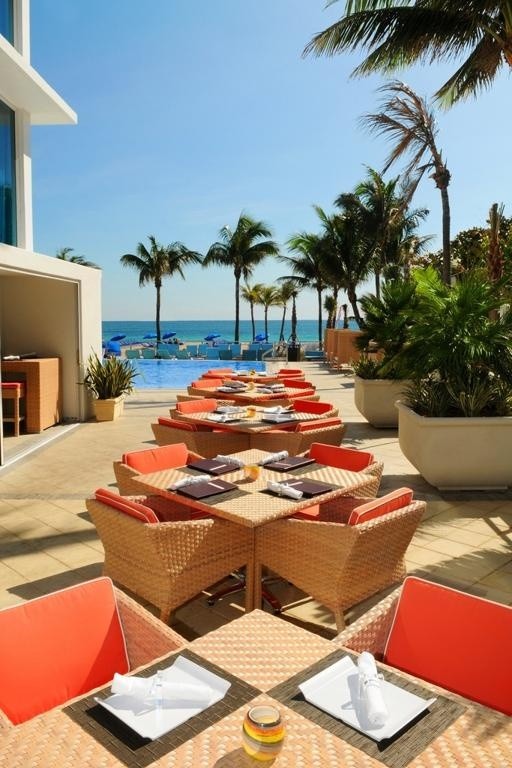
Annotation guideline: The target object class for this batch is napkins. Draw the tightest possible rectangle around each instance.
[257,450,289,465]
[267,481,302,499]
[216,454,244,466]
[168,474,211,490]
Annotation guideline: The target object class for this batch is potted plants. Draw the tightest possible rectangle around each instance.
[383,262,512,493]
[78,349,141,422]
[353,284,429,429]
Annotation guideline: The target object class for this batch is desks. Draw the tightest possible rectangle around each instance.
[131,449,377,612]
[3,355,61,436]
[0,606,512,767]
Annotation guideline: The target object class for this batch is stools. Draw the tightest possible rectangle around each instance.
[1,380,29,435]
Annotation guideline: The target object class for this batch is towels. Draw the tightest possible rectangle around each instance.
[109,668,216,708]
[354,646,385,727]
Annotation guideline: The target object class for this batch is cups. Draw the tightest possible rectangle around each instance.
[250,369,256,376]
[242,704,286,761]
[243,463,260,482]
[247,381,255,392]
[246,406,256,418]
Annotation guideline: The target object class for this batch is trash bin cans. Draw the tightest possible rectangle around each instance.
[288,344,300,361]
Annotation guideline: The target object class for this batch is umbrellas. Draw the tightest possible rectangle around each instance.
[101,331,269,360]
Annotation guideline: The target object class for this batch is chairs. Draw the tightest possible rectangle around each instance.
[0,571,190,741]
[253,485,428,632]
[99,329,301,361]
[176,379,320,407]
[281,441,385,521]
[85,486,253,624]
[112,442,208,497]
[150,398,346,460]
[198,369,306,385]
[332,574,512,717]
